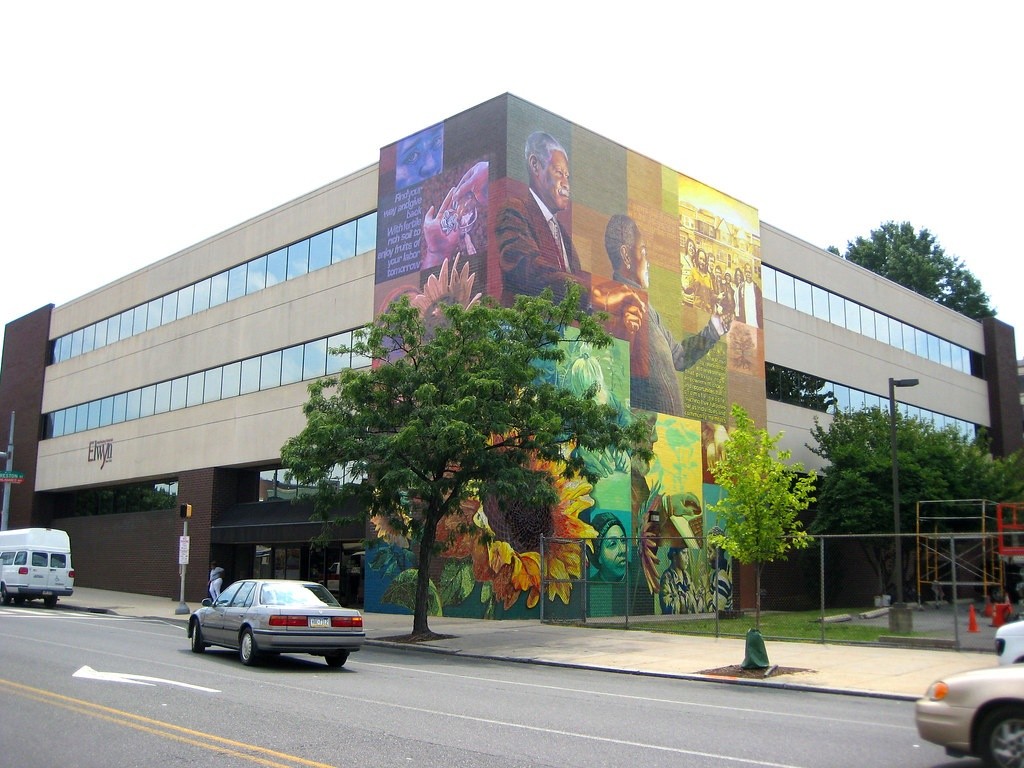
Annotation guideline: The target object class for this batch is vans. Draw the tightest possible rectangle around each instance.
[0,527,75,608]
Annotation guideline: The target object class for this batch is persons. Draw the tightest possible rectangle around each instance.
[207,561,224,601]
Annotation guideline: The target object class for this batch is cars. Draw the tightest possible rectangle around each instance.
[915,616,1024,768]
[185,577,367,668]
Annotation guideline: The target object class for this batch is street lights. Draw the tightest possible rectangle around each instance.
[888,376,920,608]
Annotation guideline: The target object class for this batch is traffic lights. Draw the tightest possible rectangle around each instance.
[180,502,193,519]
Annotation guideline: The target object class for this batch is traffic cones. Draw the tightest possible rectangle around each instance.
[966,604,981,633]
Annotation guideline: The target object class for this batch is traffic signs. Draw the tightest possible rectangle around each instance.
[0,470,27,484]
